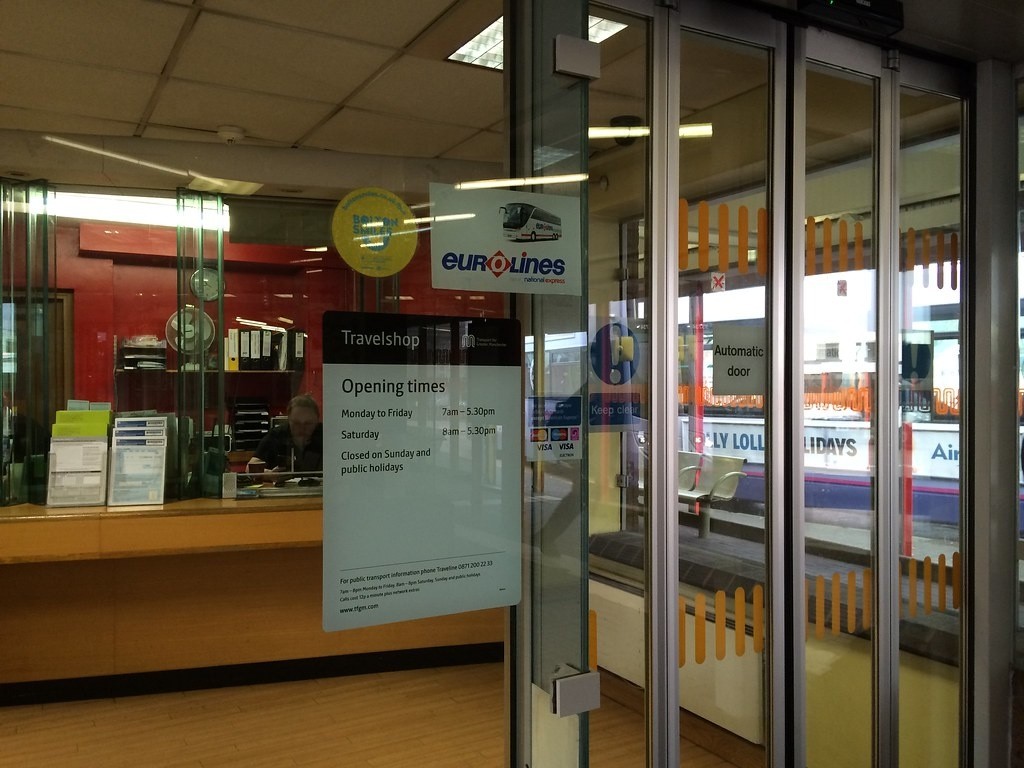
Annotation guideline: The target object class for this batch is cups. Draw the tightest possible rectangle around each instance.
[249,462,265,484]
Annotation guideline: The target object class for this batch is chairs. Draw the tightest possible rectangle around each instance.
[636,448,748,541]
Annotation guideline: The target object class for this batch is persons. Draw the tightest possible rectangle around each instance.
[244,394,323,479]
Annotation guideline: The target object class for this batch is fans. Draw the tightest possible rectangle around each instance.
[164,307,216,372]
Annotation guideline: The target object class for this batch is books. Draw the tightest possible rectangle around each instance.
[138,361,164,368]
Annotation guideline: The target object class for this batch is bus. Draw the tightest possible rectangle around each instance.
[497,201,562,242]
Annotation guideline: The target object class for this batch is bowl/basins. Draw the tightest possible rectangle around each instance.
[132,336,156,346]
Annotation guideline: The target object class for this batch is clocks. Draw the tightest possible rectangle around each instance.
[191,268,225,299]
